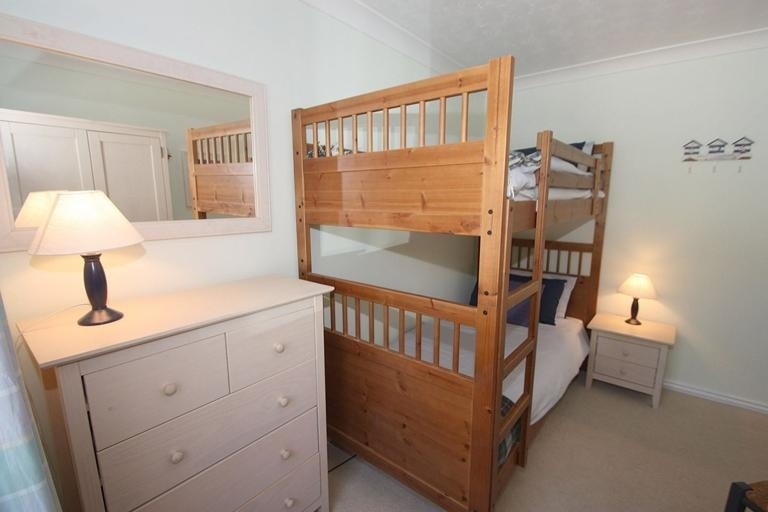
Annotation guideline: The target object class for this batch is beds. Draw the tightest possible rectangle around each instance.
[185,121,256,219]
[290,56,614,511]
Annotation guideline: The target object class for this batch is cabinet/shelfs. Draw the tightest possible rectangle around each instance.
[17,275,335,510]
[1,109,173,222]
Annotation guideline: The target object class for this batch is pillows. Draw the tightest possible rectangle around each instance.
[514,140,594,172]
[466,269,578,329]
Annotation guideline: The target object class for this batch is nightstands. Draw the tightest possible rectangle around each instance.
[584,312,676,408]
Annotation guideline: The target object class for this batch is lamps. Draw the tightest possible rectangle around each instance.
[29,190,142,327]
[617,273,655,325]
[14,192,68,231]
